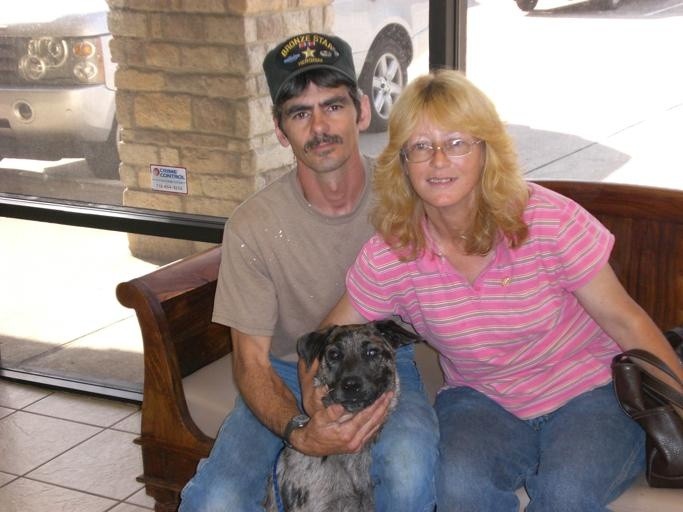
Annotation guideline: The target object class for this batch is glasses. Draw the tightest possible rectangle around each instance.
[401,137,482,164]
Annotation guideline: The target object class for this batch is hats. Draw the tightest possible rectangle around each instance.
[262,32,358,107]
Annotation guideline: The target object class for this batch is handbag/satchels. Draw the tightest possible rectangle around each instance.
[610,330,682,490]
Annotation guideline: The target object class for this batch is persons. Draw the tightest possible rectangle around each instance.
[176,31,437,509]
[296,65,682,509]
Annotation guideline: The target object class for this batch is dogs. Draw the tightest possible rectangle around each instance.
[261,319,428,512]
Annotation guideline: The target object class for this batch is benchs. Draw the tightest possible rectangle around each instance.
[116,181,682,512]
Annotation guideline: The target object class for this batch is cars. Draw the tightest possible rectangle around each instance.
[508,0,630,15]
[0,0,432,179]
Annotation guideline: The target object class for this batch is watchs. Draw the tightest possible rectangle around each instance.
[281,411,312,449]
